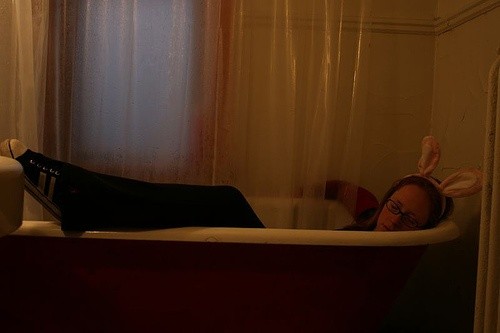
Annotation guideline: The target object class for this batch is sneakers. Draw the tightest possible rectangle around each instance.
[1,138,62,221]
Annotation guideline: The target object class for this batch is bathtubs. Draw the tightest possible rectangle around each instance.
[0,186,460,333]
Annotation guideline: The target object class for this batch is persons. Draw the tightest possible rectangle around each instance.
[0,137,483,232]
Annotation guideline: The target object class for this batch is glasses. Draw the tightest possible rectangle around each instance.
[386,198,421,227]
